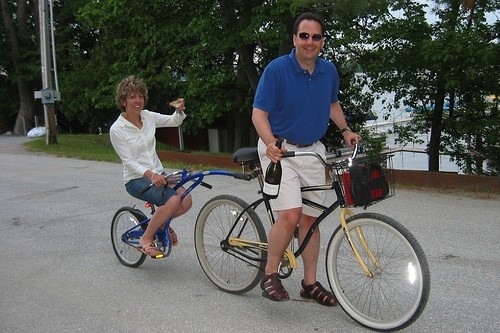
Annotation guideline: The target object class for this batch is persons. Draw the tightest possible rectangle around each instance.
[251,12,362,307]
[109,75,193,259]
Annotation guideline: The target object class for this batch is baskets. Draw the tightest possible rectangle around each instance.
[330,153,395,208]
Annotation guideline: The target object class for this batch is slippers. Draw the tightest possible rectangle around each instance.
[137,242,162,257]
[168,226,178,246]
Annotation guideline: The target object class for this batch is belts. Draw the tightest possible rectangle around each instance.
[273,135,313,148]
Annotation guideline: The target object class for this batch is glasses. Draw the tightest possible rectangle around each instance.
[298,32,323,41]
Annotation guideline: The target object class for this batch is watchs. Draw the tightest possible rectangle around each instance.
[340,126,352,134]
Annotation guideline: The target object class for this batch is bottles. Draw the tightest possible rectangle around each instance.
[262,139,282,199]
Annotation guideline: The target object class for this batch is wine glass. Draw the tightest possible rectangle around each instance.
[169,100,183,119]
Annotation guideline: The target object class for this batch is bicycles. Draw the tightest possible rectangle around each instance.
[110,139,432,333]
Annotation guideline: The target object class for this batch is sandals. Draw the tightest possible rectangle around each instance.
[300,279,337,306]
[260,273,289,302]
